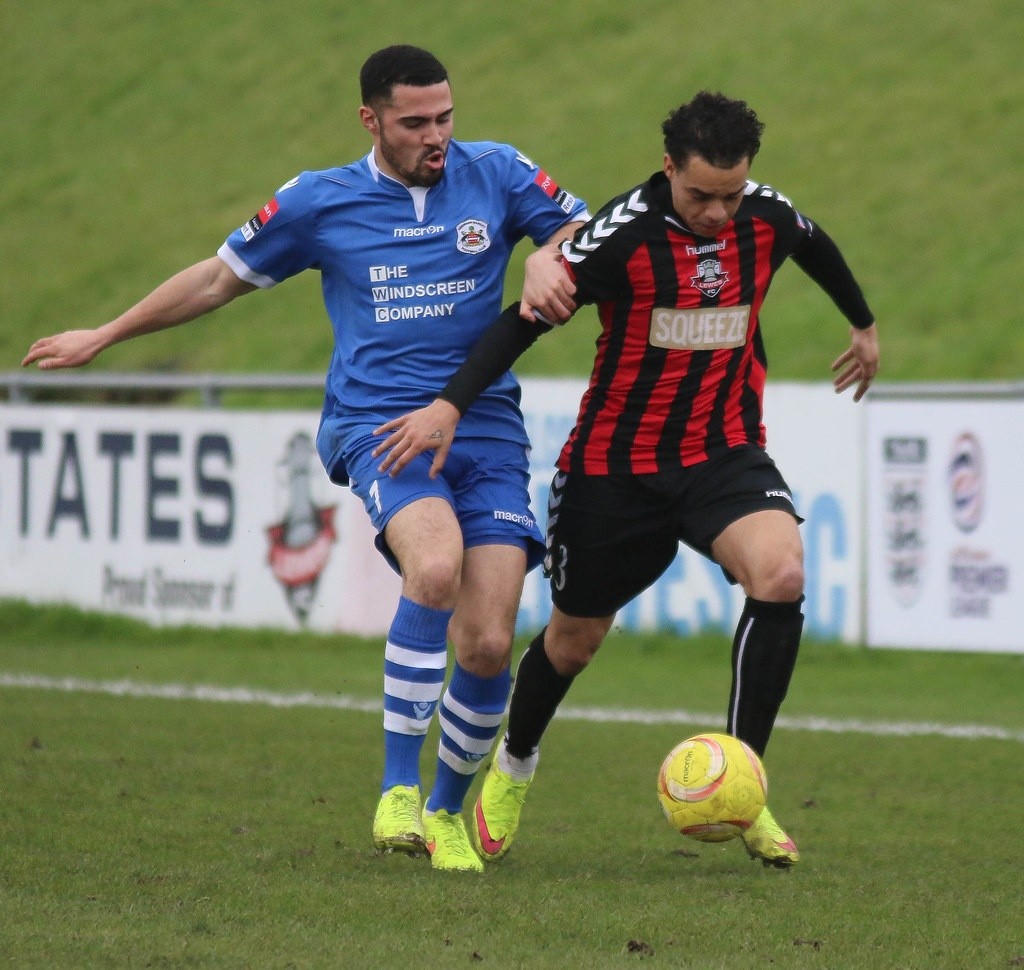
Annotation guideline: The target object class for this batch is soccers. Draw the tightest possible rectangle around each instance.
[657,732,768,843]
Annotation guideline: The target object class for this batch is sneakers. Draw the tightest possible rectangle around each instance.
[473,733,534,865]
[748,807,801,868]
[422,796,486,875]
[372,784,426,857]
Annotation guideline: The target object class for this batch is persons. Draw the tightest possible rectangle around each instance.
[21,45,592,871]
[371,89,879,870]
